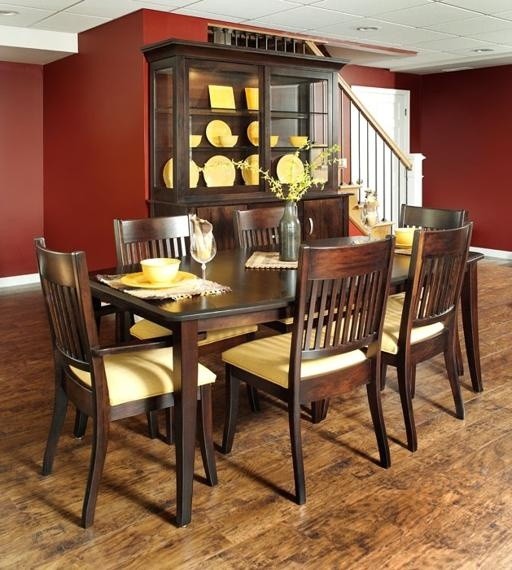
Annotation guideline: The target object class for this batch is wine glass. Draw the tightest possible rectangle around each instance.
[190,232,218,279]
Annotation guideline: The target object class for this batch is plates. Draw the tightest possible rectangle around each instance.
[205,120,232,147]
[121,271,197,289]
[247,121,259,147]
[163,157,200,188]
[241,155,259,186]
[276,155,304,184]
[204,156,235,188]
[393,244,413,249]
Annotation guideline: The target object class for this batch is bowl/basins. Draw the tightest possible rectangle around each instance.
[395,228,414,244]
[270,136,278,146]
[141,258,182,283]
[288,137,308,147]
[231,136,238,146]
[190,134,202,147]
